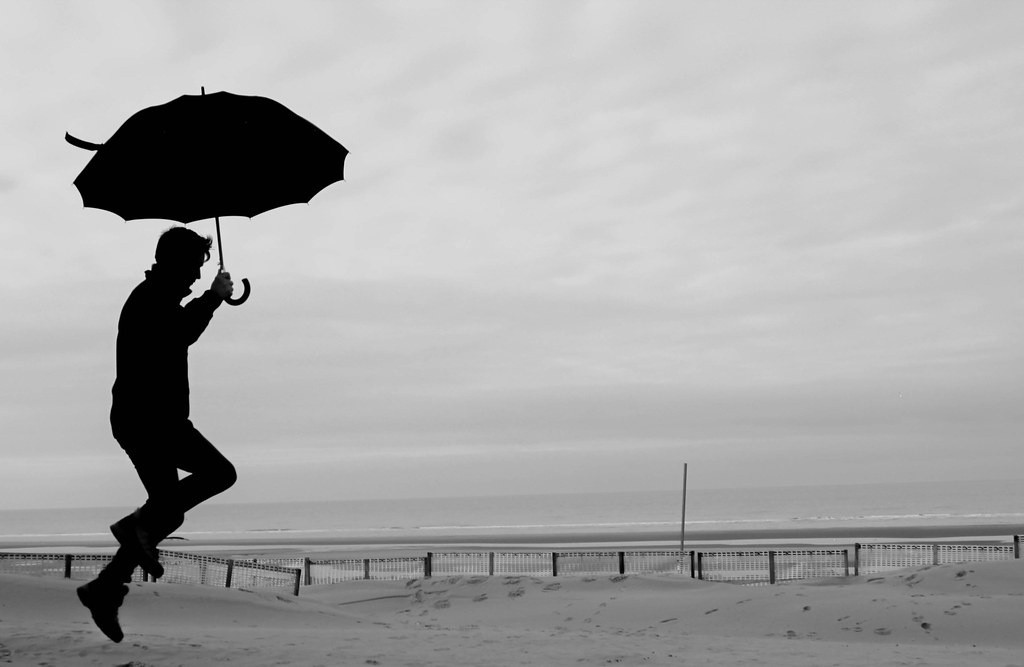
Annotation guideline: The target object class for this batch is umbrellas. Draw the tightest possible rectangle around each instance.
[65,86,349,307]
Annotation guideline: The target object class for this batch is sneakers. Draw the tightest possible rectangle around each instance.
[77,574,129,643]
[110,510,165,579]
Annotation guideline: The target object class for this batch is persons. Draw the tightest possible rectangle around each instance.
[75,227,237,643]
